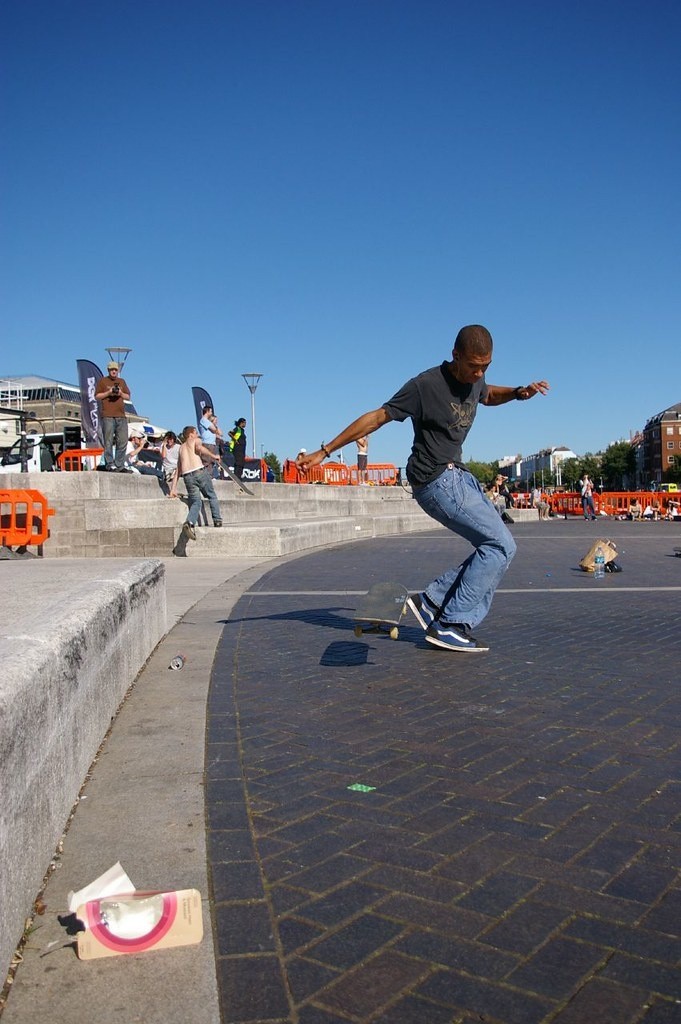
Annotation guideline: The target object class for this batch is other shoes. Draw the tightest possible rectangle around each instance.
[120,468,133,473]
[214,521,222,527]
[183,521,196,540]
[109,465,119,472]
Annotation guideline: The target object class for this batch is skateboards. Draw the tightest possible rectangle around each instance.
[215,459,255,496]
[351,582,410,639]
[672,546,681,558]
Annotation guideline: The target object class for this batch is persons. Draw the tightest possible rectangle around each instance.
[295,448,308,476]
[160,431,182,497]
[483,474,515,517]
[228,418,247,483]
[179,426,223,540]
[199,406,223,480]
[396,471,402,486]
[94,361,134,474]
[530,487,564,522]
[295,325,549,652]
[356,434,371,486]
[124,431,170,497]
[579,473,597,522]
[627,498,681,522]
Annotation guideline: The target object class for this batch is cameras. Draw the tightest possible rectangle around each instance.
[587,476,591,480]
[112,383,120,393]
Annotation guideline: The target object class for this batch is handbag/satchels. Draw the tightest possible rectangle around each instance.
[578,540,618,572]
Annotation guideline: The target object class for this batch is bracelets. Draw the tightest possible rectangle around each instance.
[321,444,331,458]
[513,386,524,400]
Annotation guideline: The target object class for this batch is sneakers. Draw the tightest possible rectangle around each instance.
[425,620,489,652]
[406,593,441,631]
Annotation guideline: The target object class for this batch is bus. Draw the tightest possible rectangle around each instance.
[655,482,678,493]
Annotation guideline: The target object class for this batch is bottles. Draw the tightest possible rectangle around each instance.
[594,547,605,579]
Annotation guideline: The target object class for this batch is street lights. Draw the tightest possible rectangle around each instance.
[104,347,132,378]
[43,386,62,433]
[241,373,264,459]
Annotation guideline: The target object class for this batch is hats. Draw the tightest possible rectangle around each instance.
[108,362,118,369]
[301,449,306,453]
[497,474,503,479]
[130,431,143,437]
